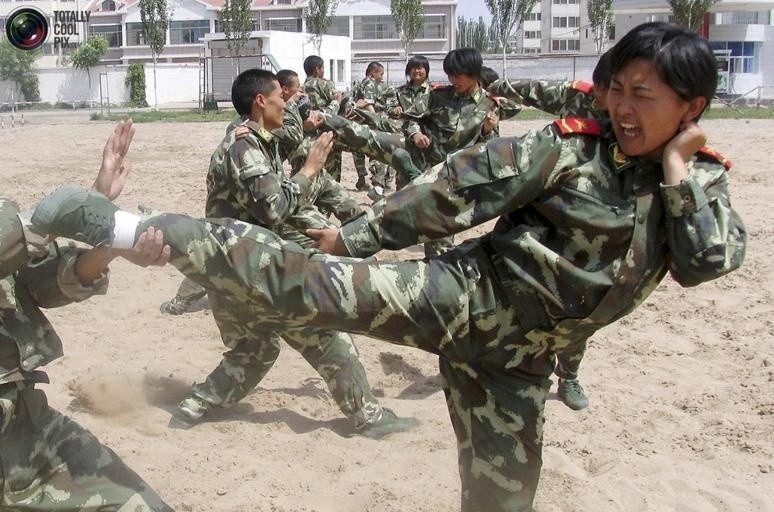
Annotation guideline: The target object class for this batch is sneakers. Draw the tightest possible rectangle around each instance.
[28,183,122,250]
[362,407,419,437]
[298,95,311,119]
[557,377,589,409]
[160,295,186,315]
[171,394,208,429]
[339,96,350,115]
[355,179,393,202]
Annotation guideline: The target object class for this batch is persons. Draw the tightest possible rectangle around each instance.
[164,67,425,442]
[156,51,523,318]
[0,118,174,512]
[30,19,747,512]
[488,45,613,410]
